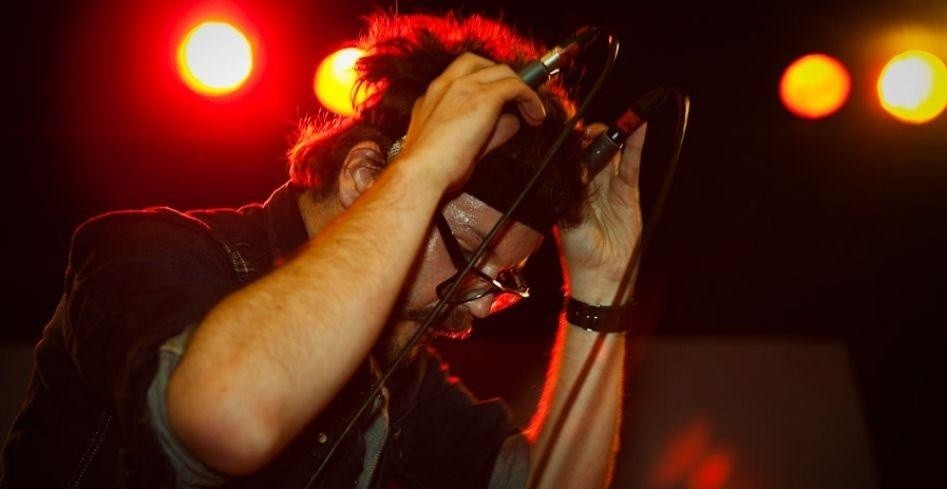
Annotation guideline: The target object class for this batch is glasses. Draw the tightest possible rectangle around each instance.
[361,151,528,322]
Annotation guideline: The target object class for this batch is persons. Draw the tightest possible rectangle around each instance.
[0,7,651,489]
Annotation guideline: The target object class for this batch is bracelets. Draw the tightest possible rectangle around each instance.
[560,295,638,336]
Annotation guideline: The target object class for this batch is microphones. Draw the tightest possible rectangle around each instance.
[381,22,612,188]
[584,73,672,186]
